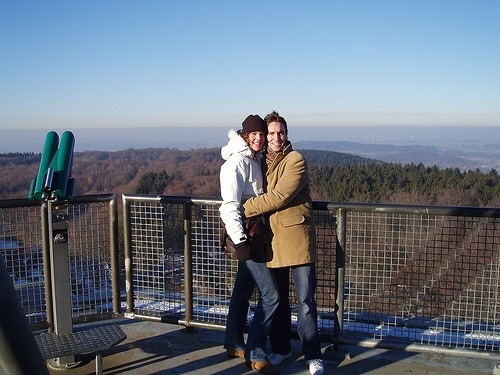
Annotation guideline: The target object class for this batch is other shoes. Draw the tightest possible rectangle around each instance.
[227,346,246,359]
[245,357,280,375]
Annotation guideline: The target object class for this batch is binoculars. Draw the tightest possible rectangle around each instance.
[29,130,76,201]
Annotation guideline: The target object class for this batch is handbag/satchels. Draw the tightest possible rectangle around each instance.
[220,213,264,259]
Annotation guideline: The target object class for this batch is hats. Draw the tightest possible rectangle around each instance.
[242,115,267,134]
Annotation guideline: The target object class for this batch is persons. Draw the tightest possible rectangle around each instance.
[220,111,325,375]
[218,113,279,375]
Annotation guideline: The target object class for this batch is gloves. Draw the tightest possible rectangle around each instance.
[235,242,252,263]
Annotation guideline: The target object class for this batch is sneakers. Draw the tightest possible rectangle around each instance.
[305,358,324,375]
[266,350,292,365]
